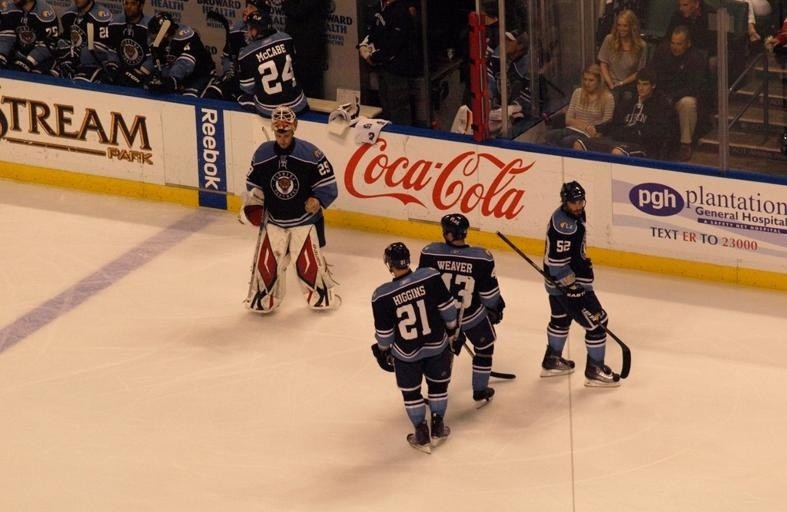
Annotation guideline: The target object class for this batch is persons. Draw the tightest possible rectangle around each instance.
[539,179,623,388]
[544,2,787,162]
[241,105,339,316]
[206,0,339,119]
[358,2,552,143]
[1,1,224,104]
[416,213,508,406]
[368,239,466,454]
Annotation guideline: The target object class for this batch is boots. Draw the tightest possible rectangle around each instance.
[431,412,449,438]
[585,354,619,382]
[406,420,431,445]
[542,344,575,370]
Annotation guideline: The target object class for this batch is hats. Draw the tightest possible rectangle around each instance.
[505,29,527,41]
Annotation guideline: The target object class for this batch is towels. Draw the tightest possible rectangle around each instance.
[351,116,391,144]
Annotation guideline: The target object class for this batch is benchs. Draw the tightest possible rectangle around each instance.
[303,93,384,123]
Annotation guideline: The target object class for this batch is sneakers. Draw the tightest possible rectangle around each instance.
[473,388,494,401]
[680,143,693,162]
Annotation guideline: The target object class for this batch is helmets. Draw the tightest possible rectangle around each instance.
[271,107,297,134]
[148,12,177,36]
[384,242,411,270]
[441,214,468,240]
[246,0,273,38]
[561,181,585,202]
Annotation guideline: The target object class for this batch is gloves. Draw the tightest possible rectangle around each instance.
[371,343,394,373]
[567,285,586,313]
[150,76,177,93]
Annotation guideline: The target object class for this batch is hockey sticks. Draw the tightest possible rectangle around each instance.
[463,343,516,379]
[496,233,631,379]
[87,22,114,84]
[208,9,240,98]
[153,20,171,92]
